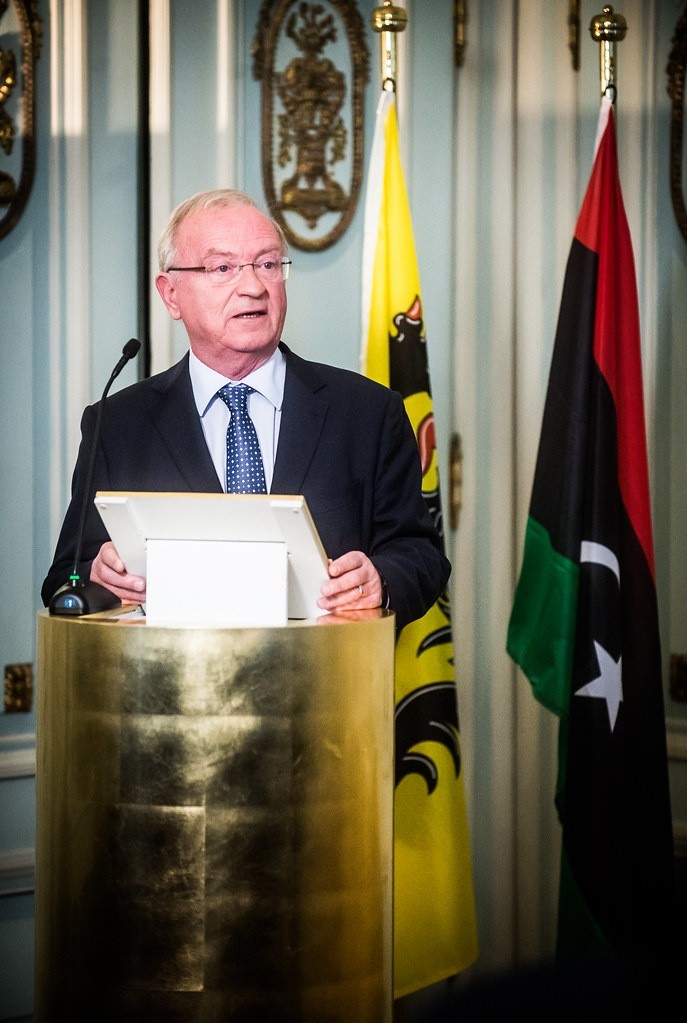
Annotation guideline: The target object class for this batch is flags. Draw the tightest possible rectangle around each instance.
[501,92,687,1022]
[359,91,485,1001]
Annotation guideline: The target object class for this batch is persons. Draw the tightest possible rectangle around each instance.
[39,188,451,617]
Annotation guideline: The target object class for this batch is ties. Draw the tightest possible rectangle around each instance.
[217,383,267,495]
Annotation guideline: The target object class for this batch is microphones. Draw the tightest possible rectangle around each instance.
[48,338,141,617]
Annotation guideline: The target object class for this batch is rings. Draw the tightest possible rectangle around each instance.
[359,584,365,598]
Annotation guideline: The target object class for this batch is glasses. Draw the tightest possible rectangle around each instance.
[167,257,291,282]
